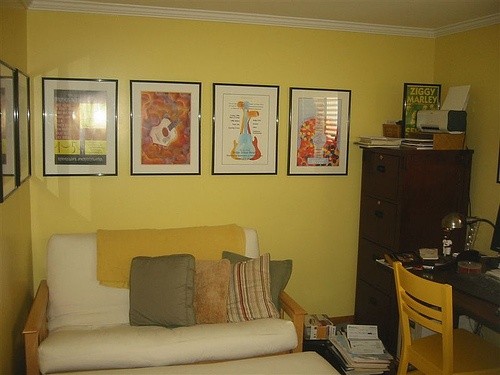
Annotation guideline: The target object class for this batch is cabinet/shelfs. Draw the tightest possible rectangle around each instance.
[354,146,474,358]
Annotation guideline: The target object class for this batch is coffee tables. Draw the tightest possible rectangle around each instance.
[73,350,343,375]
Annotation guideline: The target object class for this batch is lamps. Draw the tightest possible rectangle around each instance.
[440,214,497,231]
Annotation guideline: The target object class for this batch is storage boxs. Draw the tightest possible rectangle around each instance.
[382,123,402,138]
[304,313,335,340]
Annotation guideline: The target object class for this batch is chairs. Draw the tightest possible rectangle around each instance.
[393,261,500,375]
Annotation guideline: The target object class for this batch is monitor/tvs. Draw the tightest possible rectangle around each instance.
[490,204,500,253]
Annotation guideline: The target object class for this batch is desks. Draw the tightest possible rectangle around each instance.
[405,256,500,334]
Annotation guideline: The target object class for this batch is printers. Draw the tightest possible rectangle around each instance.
[416,96,470,135]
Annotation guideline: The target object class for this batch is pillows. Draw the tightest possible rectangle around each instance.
[225,251,281,323]
[128,253,196,327]
[222,250,293,318]
[193,259,231,325]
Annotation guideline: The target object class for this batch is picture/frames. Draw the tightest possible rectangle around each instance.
[0,59,18,204]
[16,68,31,187]
[130,80,201,176]
[41,77,119,177]
[287,88,351,176]
[211,83,280,175]
[402,83,441,138]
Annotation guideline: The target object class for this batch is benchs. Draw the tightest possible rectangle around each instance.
[22,223,309,375]
